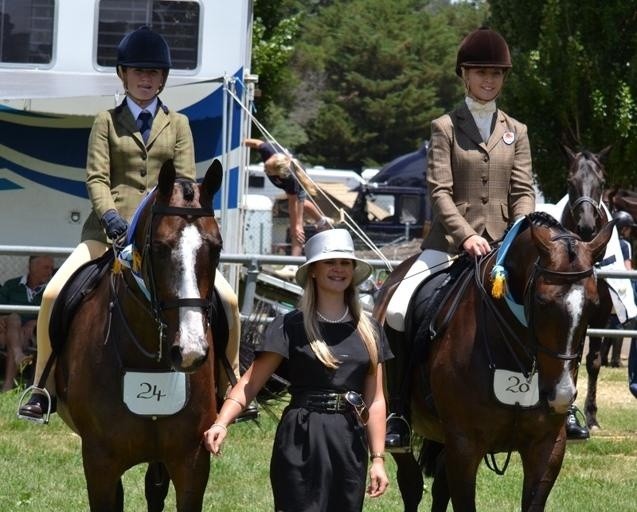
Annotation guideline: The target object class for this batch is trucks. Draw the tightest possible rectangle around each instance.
[243,138,431,256]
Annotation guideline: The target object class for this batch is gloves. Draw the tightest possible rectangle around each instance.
[102,212,129,239]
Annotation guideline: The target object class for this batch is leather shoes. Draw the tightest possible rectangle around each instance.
[385,411,411,449]
[17,395,57,419]
[565,407,591,440]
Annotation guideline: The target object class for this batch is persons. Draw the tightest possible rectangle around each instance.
[599,211,637,366]
[203,226,389,512]
[386,32,591,448]
[237,136,334,277]
[0,252,55,394]
[20,26,259,419]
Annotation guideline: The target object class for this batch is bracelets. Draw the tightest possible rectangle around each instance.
[211,421,228,431]
[224,397,247,411]
[369,452,385,461]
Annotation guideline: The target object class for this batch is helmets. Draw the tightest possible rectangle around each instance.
[614,208,636,230]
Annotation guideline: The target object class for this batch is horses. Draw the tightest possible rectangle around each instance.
[372,212,620,512]
[560,143,615,434]
[48,158,291,512]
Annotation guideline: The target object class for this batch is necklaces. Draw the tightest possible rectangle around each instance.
[313,302,349,324]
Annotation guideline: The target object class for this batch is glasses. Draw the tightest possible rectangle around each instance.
[344,390,370,427]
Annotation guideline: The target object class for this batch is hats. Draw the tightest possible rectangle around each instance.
[453,27,513,76]
[294,226,373,287]
[115,23,172,70]
